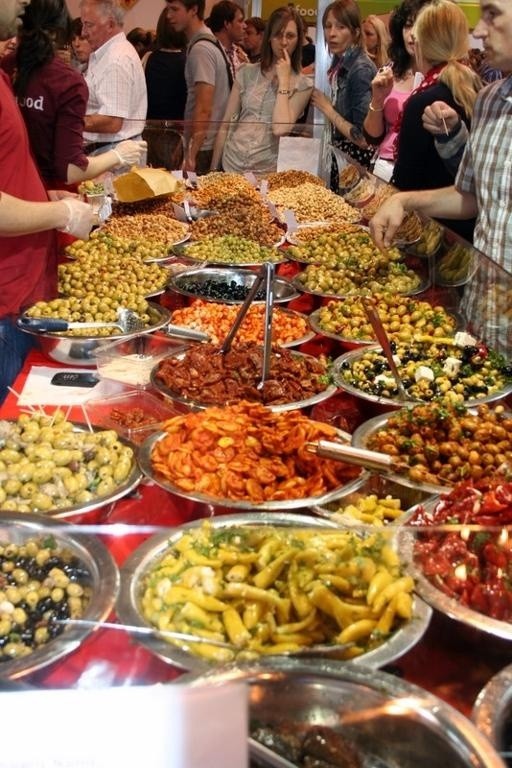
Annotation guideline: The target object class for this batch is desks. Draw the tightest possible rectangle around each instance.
[1,162,512,763]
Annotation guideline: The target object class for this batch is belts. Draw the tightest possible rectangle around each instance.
[84,142,110,154]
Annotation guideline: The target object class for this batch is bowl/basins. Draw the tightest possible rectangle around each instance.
[150,341,337,413]
[0,219,511,310]
[331,342,511,408]
[170,306,319,351]
[398,493,511,640]
[85,391,183,443]
[15,300,173,366]
[88,331,187,389]
[112,512,434,672]
[305,304,461,352]
[470,663,511,768]
[352,408,511,497]
[137,420,371,513]
[166,656,505,768]
[1,509,121,680]
[0,416,145,518]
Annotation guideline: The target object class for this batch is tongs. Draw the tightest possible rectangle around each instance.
[221,261,275,396]
